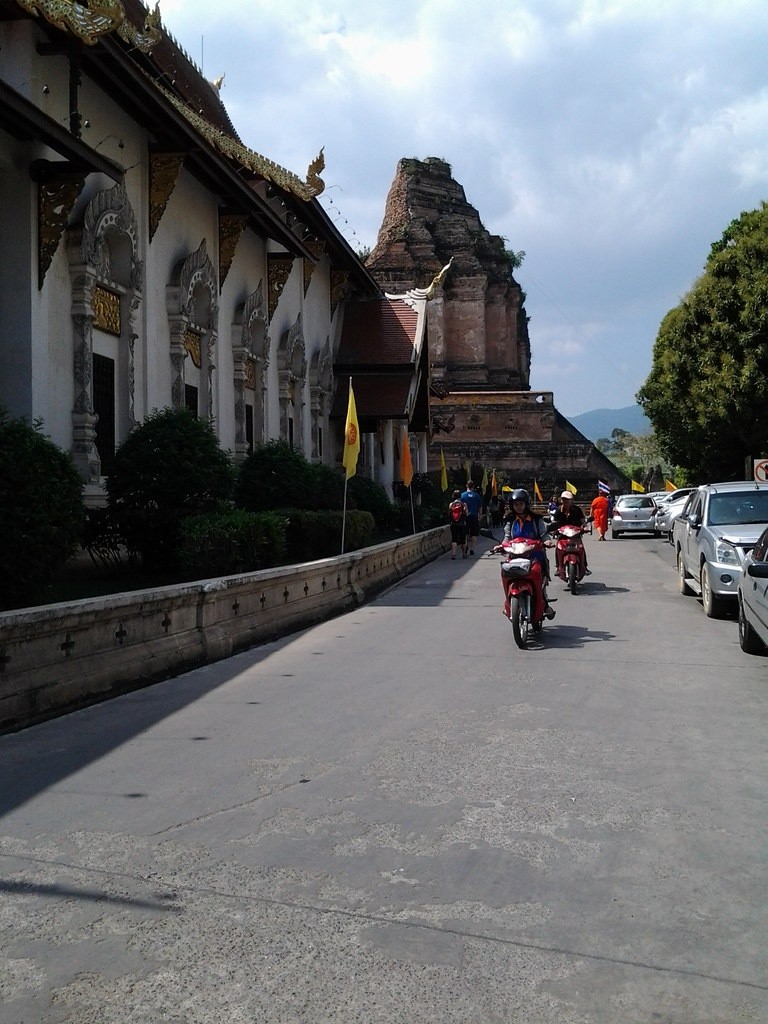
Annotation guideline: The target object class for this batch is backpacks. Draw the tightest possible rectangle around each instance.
[451,499,466,521]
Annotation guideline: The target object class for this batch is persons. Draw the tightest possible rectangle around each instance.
[449,490,471,560]
[547,495,561,522]
[461,480,482,556]
[589,489,613,541]
[494,489,556,620]
[548,491,592,576]
[489,494,505,528]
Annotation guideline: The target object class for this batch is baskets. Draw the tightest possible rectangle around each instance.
[500,560,531,576]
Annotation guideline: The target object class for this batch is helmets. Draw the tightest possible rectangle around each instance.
[509,489,532,514]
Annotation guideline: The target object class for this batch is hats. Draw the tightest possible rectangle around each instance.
[561,491,573,499]
[452,490,461,499]
[497,495,502,499]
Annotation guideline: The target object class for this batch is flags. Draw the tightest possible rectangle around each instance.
[632,480,645,493]
[466,462,472,483]
[535,482,543,501]
[481,469,488,495]
[343,386,360,480]
[401,432,414,487]
[598,481,610,493]
[492,471,497,496]
[666,479,678,492]
[566,481,577,495]
[440,451,448,492]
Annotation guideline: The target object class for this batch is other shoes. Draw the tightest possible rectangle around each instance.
[463,554,469,558]
[496,526,499,528]
[545,606,556,620]
[554,569,560,576]
[451,555,456,560]
[465,546,469,553]
[584,568,592,575]
[493,527,495,528]
[470,550,474,555]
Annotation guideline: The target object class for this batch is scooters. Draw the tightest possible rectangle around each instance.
[479,521,560,648]
[542,515,595,595]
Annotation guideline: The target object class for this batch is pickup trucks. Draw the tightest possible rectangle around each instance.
[672,480,768,620]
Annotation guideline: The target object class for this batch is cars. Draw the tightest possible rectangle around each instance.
[734,524,767,655]
[609,494,662,539]
[635,487,730,532]
